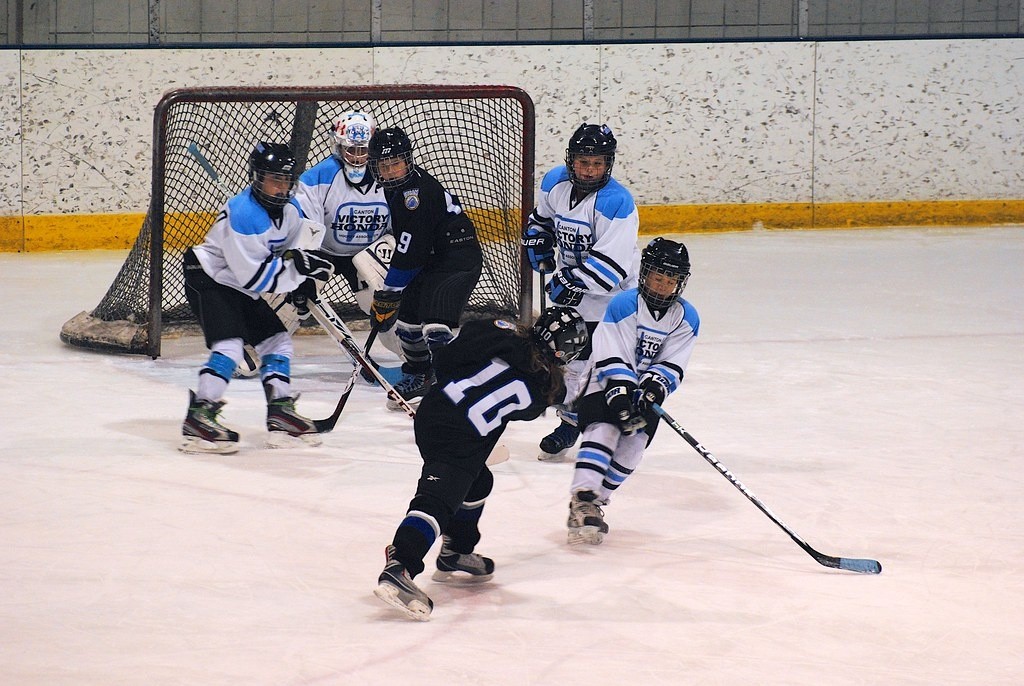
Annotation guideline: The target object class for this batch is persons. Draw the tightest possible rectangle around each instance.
[232,110,408,377]
[520,123,642,461]
[366,126,483,412]
[176,142,331,455]
[374,307,589,623]
[567,237,700,548]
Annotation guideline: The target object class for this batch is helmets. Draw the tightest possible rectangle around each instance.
[564,123,617,193]
[637,236,690,308]
[529,305,588,367]
[367,126,413,188]
[247,142,299,219]
[329,110,376,185]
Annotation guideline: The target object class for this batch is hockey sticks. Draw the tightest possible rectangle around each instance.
[652,402,882,575]
[306,299,509,466]
[539,262,579,427]
[319,298,403,386]
[313,325,378,433]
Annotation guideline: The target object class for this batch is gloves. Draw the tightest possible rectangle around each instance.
[544,266,589,307]
[633,372,671,419]
[603,380,641,436]
[370,290,404,333]
[289,278,322,316]
[284,248,335,282]
[522,232,557,274]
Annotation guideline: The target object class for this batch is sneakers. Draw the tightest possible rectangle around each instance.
[178,389,240,453]
[264,383,322,448]
[374,545,433,621]
[567,488,603,546]
[433,534,495,584]
[589,498,610,545]
[386,362,436,408]
[537,414,581,460]
